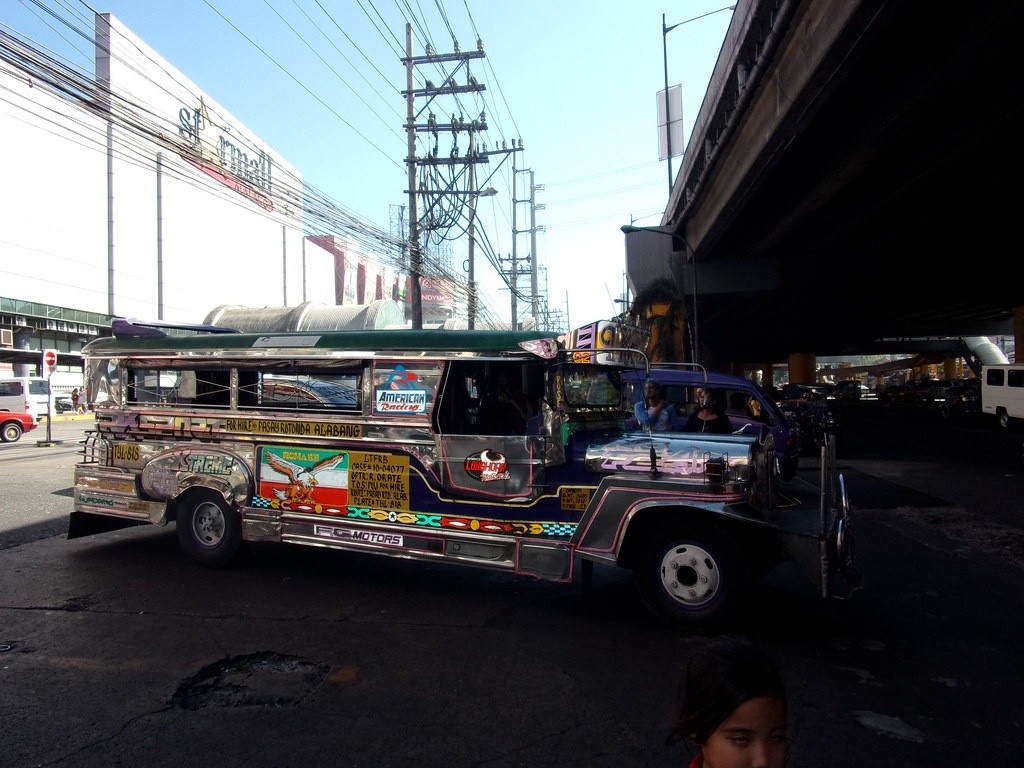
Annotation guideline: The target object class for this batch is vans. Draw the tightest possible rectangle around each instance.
[981,363,1024,429]
[620,367,799,485]
[0,377,56,422]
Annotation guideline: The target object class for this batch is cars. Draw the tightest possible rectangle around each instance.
[775,398,845,449]
[263,377,356,409]
[879,385,915,409]
[0,411,39,442]
[911,382,949,409]
[946,377,981,412]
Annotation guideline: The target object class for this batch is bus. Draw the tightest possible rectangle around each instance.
[839,380,861,401]
[65,329,855,622]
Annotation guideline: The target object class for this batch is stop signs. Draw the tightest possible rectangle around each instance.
[45,352,56,365]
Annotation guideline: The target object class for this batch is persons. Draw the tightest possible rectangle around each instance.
[634,381,681,432]
[665,632,790,768]
[72,385,90,415]
[685,388,733,435]
